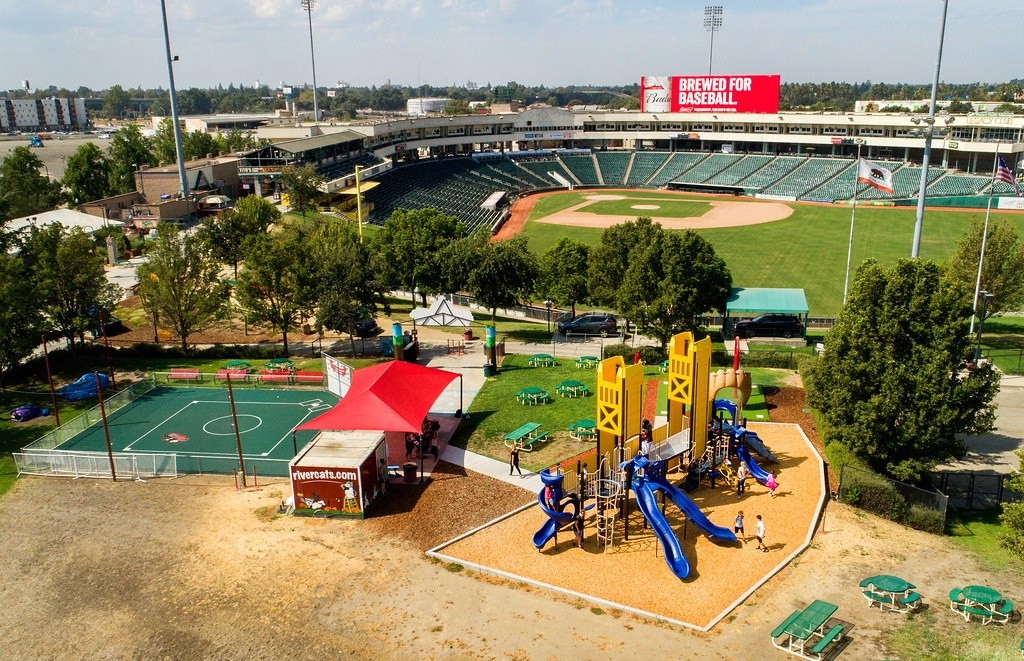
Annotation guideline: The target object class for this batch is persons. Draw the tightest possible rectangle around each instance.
[509,445,521,476]
[634,350,641,365]
[765,470,778,496]
[641,418,652,459]
[724,457,732,467]
[545,484,556,512]
[733,510,745,542]
[573,510,585,548]
[756,515,768,552]
[737,461,748,499]
[405,430,440,461]
[712,417,720,432]
[579,463,588,483]
[381,459,389,494]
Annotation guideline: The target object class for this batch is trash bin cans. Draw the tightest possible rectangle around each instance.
[464,328,472,340]
[403,461,417,482]
[483,363,493,377]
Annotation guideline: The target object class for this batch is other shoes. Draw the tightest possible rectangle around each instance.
[737,495,742,500]
[762,548,768,552]
[508,474,512,476]
[756,546,761,550]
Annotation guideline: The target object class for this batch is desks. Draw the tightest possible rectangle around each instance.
[962,585,1003,620]
[576,419,596,443]
[871,574,910,610]
[533,353,551,368]
[227,359,245,366]
[561,380,582,399]
[507,422,542,448]
[581,356,597,368]
[663,360,669,371]
[523,387,545,406]
[783,600,839,655]
[270,357,288,364]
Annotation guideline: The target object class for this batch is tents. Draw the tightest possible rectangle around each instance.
[292,359,463,483]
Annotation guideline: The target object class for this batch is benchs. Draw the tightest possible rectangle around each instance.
[388,466,400,477]
[859,577,921,612]
[949,588,1013,625]
[167,368,204,384]
[264,360,294,370]
[504,356,666,452]
[221,362,252,369]
[212,369,250,385]
[771,610,844,661]
[256,370,326,387]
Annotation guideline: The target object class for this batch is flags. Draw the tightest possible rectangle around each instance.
[995,154,1021,196]
[857,157,893,194]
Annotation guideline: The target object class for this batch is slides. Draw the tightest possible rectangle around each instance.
[636,484,691,578]
[532,468,572,549]
[656,477,738,543]
[738,446,771,484]
